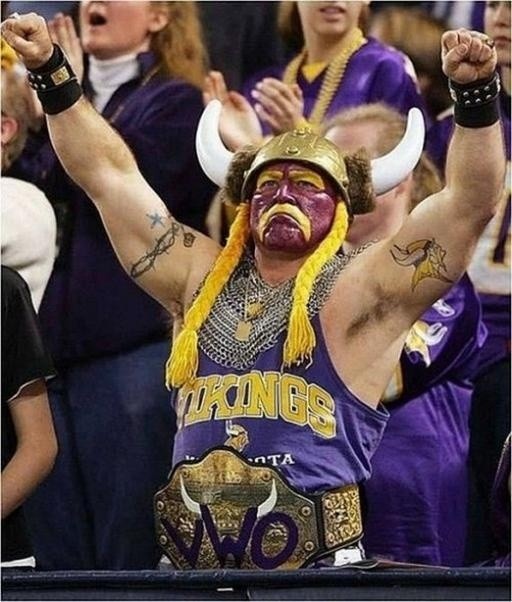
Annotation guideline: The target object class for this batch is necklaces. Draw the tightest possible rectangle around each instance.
[233,263,295,347]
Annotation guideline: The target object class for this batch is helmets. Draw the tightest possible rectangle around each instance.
[240,125,350,204]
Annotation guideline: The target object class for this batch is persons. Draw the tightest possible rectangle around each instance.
[425,2,511,568]
[22,1,220,570]
[1,9,509,569]
[200,1,428,137]
[2,262,60,572]
[199,66,490,567]
[1,62,59,315]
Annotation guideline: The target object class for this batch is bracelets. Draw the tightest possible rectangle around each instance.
[25,43,85,115]
[447,70,503,130]
